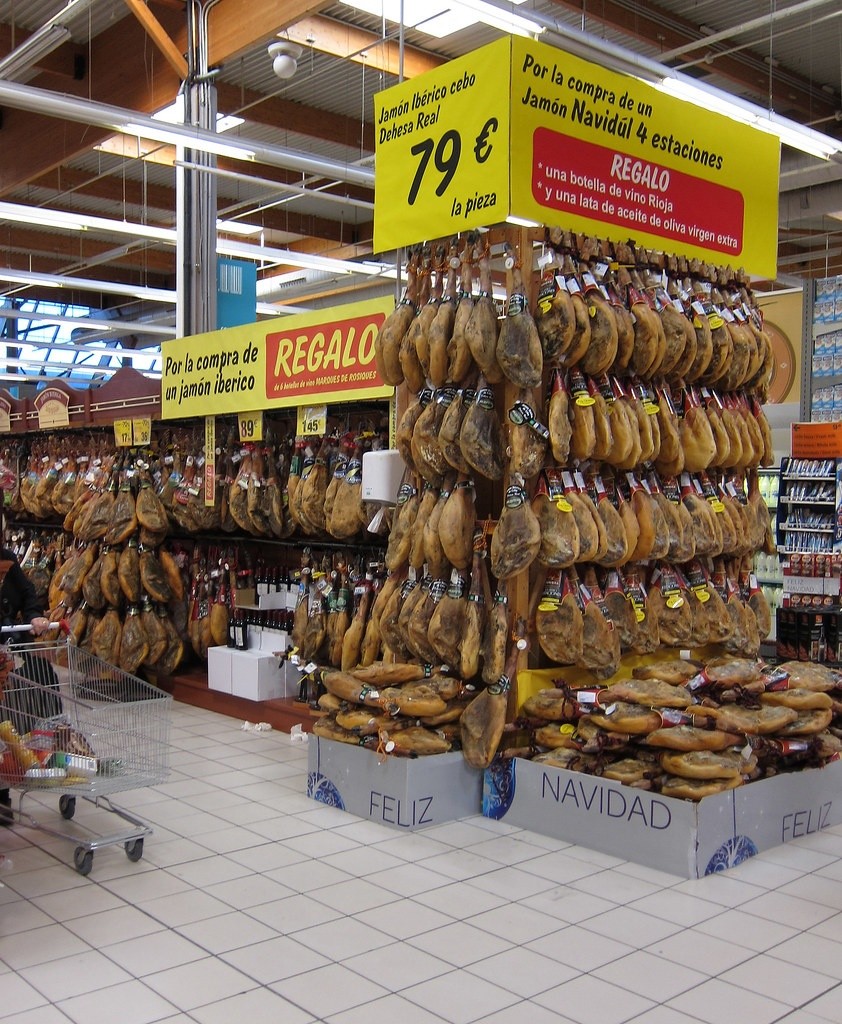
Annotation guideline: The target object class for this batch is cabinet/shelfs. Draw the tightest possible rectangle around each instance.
[749,468,784,642]
[776,422,842,564]
[799,277,842,423]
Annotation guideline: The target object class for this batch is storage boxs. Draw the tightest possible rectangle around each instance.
[208,645,249,694]
[799,610,827,662]
[776,608,802,657]
[787,609,810,660]
[307,733,479,833]
[56,751,98,776]
[822,408,833,422]
[811,389,822,409]
[286,584,300,609]
[811,408,822,423]
[828,613,842,664]
[482,754,842,880]
[822,386,833,409]
[232,650,285,702]
[235,584,286,610]
[812,275,842,377]
[833,386,842,422]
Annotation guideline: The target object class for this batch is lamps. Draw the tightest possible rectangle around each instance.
[0,0,842,384]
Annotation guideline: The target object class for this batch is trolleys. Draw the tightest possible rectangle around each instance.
[0,618,173,876]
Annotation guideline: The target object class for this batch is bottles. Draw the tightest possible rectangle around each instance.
[254,564,289,603]
[246,609,297,634]
[743,474,781,616]
[226,608,248,651]
[776,612,842,662]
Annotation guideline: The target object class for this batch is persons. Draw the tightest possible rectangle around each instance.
[0,487,121,828]
